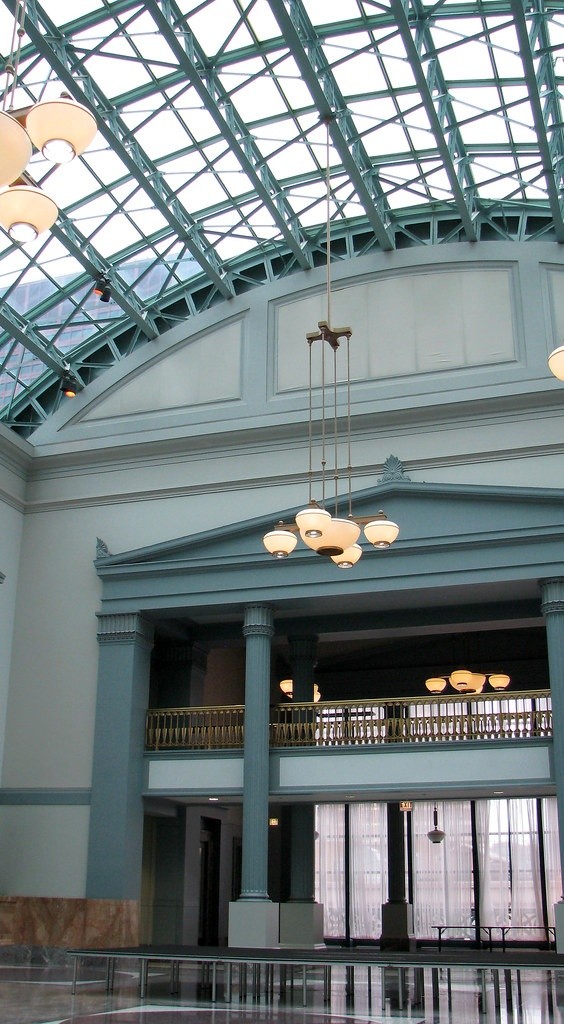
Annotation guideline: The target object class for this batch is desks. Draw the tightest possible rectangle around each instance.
[73,940,563,1008]
[430,918,558,950]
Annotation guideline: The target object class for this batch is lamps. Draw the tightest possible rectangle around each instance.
[101,286,111,303]
[279,680,321,703]
[60,379,77,397]
[546,345,564,381]
[424,664,510,696]
[93,271,111,296]
[259,113,399,569]
[427,807,446,846]
[0,1,98,242]
